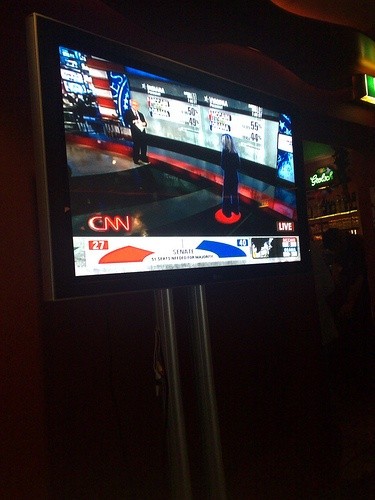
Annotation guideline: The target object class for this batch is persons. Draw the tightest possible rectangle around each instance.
[124,99,149,165]
[218,133,240,218]
[322,227,367,320]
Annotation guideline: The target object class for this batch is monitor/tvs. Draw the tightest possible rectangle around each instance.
[26,12,313,303]
